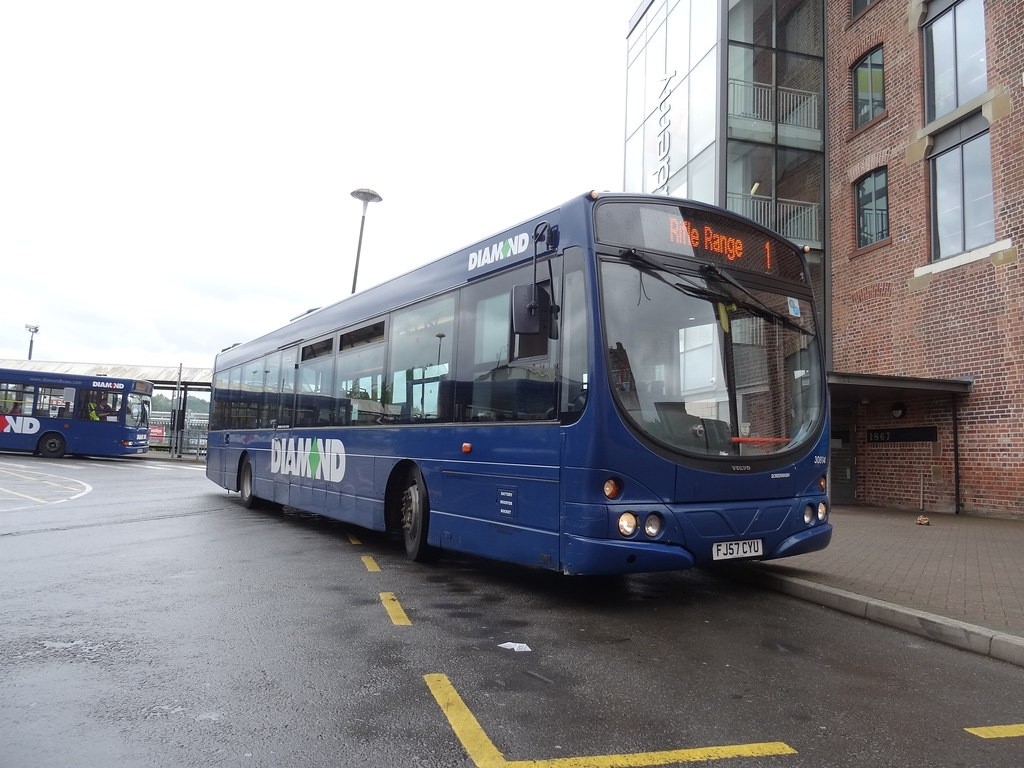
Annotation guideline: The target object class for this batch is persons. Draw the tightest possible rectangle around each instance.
[95,398,114,421]
[116,398,133,417]
[0,405,5,412]
[8,401,21,415]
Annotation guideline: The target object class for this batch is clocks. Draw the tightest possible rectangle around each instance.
[891,401,905,418]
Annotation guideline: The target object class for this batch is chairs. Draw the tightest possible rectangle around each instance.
[1,402,101,421]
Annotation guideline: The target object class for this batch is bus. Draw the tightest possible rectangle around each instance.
[205,188,837,611]
[0,368,155,470]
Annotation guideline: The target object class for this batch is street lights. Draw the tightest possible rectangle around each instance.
[22,323,39,360]
[347,187,384,298]
